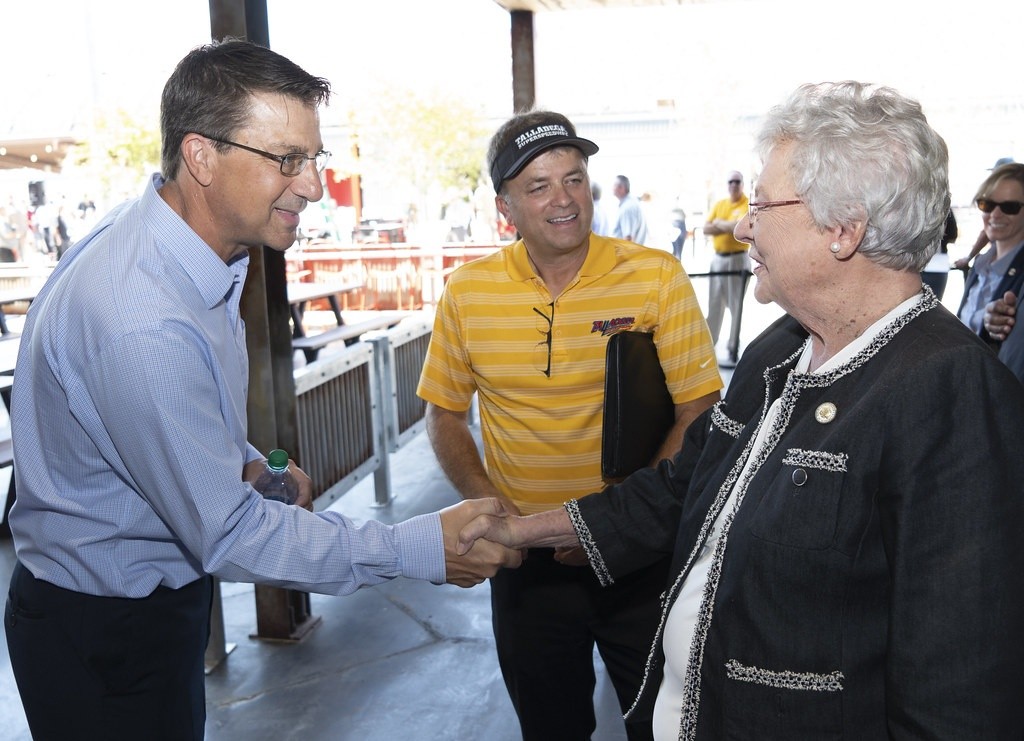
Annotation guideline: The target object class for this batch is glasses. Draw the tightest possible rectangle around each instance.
[976,198,1024,215]
[748,200,800,228]
[183,129,333,177]
[533,301,554,377]
[729,180,740,184]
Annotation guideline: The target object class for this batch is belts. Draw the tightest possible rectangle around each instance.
[720,250,745,256]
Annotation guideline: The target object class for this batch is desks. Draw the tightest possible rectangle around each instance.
[0,287,41,396]
[288,282,363,363]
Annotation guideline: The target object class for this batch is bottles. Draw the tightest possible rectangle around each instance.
[254,450,298,505]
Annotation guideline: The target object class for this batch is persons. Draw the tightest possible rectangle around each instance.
[591,158,1024,384]
[416,111,725,741]
[4,38,524,741]
[453,79,1024,741]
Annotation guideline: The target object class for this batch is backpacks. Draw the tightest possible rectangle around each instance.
[941,209,957,253]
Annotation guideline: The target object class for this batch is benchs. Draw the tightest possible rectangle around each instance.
[291,315,402,364]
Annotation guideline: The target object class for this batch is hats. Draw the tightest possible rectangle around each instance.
[490,122,599,194]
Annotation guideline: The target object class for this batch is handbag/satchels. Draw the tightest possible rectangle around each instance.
[601,330,675,479]
